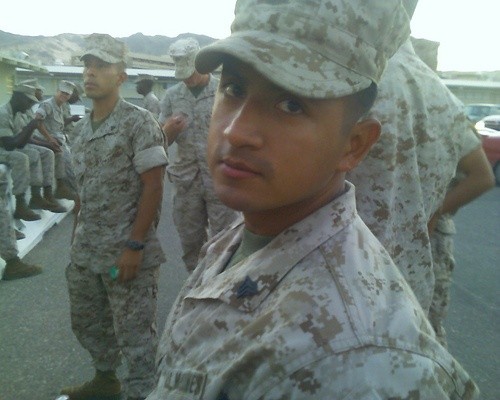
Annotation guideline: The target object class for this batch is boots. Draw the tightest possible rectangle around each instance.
[11,177,80,241]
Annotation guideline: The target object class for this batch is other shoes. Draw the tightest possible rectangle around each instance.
[3,256,42,279]
[59,368,123,399]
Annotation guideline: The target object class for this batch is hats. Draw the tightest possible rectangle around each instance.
[20,79,43,90]
[14,84,39,103]
[80,33,128,68]
[169,37,200,80]
[58,80,76,95]
[72,81,84,101]
[195,0,419,98]
[134,74,154,83]
[410,36,440,72]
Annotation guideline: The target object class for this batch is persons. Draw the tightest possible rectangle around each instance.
[158,37,242,275]
[344,35,496,341]
[145,0,482,400]
[0,78,92,281]
[60,33,171,400]
[134,73,161,121]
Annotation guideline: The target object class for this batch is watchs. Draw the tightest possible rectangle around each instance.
[123,239,145,251]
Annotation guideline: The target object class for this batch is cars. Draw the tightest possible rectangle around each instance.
[476,113,500,187]
